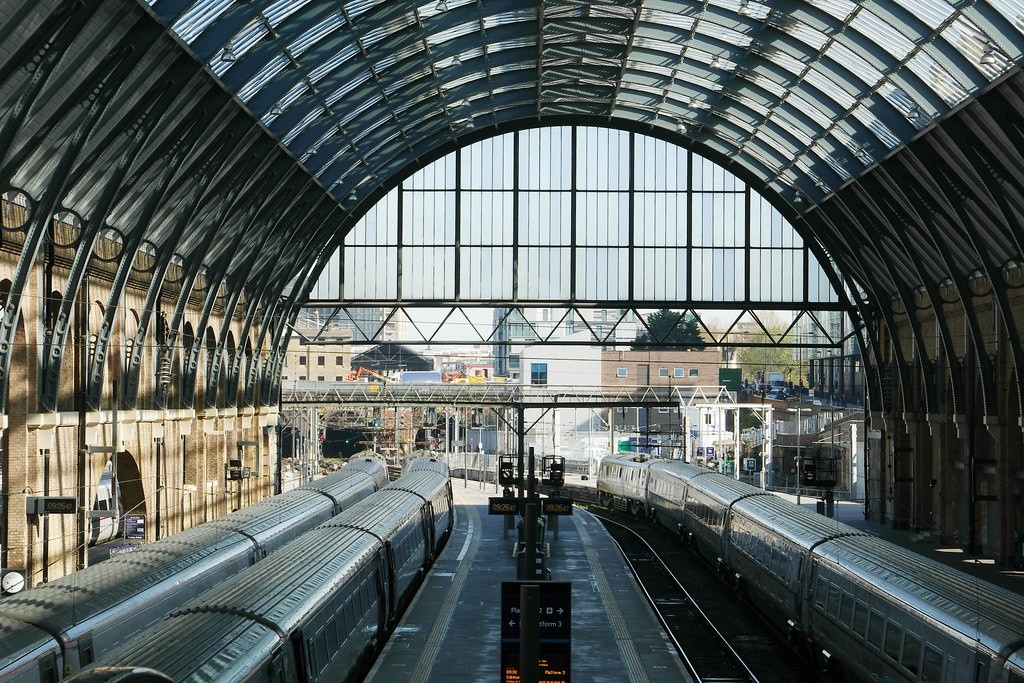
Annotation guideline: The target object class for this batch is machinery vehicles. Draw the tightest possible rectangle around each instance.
[442,371,467,383]
[346,367,395,383]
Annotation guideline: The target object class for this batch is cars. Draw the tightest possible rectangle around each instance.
[767,390,779,398]
[827,395,845,407]
[806,400,822,406]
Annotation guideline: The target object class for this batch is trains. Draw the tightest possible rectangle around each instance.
[597,452,1024,683]
[0,451,390,682]
[58,457,453,683]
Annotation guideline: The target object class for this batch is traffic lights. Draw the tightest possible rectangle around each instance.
[319,429,323,439]
[375,422,378,427]
[799,380,803,388]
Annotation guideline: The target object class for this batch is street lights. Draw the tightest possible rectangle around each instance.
[828,350,832,403]
[819,408,847,516]
[800,332,814,399]
[786,408,812,505]
[817,351,821,402]
[744,331,781,396]
